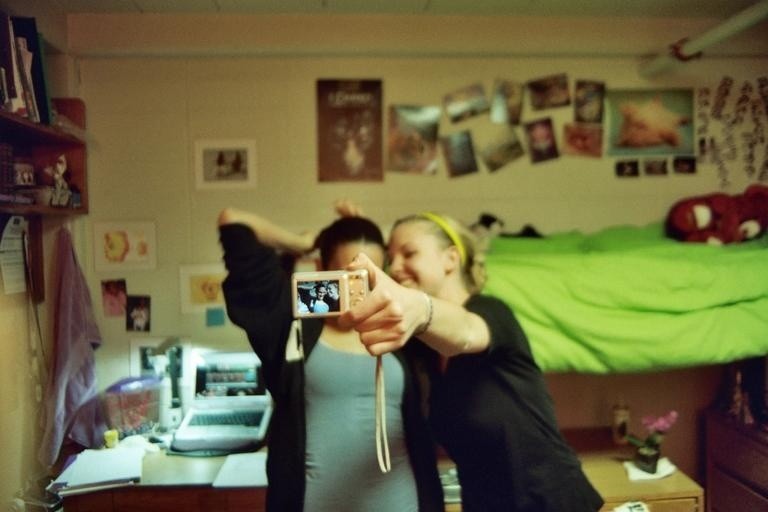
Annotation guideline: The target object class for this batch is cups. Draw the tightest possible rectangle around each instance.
[104,429,118,448]
[612,403,630,444]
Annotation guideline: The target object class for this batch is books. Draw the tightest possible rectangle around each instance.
[0,10,42,124]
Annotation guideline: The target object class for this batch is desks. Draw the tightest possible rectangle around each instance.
[61,484,267,510]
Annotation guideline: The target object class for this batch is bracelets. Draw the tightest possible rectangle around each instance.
[414,291,434,338]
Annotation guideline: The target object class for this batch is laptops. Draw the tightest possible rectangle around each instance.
[175,352,274,440]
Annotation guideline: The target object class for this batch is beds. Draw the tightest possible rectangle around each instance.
[375,184,766,374]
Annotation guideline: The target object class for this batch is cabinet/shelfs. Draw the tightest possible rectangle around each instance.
[0,94,90,214]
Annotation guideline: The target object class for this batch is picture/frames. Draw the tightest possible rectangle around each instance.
[192,139,256,191]
[603,86,698,159]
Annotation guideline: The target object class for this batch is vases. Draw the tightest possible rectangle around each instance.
[638,445,660,474]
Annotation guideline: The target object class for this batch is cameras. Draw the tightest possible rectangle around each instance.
[292,270,370,318]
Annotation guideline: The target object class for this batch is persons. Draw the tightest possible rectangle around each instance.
[333,200,604,511]
[298,281,339,314]
[130,299,149,331]
[216,206,447,512]
[102,281,127,316]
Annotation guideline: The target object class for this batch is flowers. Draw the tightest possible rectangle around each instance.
[641,412,676,434]
